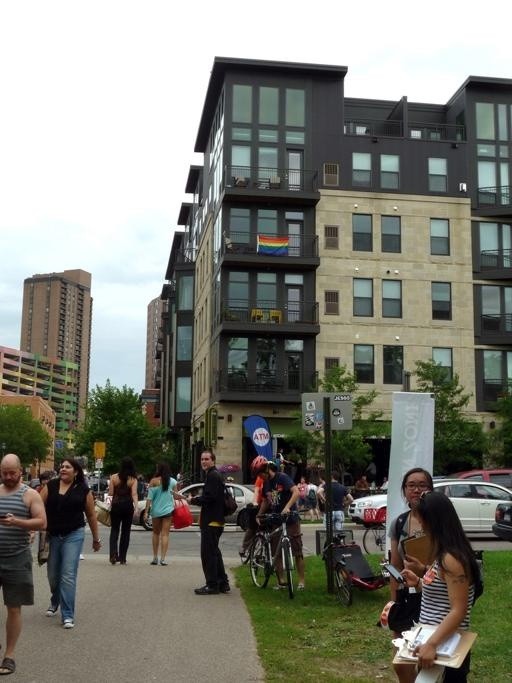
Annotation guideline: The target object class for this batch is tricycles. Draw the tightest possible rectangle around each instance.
[325,532,392,605]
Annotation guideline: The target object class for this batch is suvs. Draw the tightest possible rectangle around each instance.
[348,477,447,529]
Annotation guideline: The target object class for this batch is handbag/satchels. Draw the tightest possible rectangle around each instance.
[237,503,259,530]
[214,490,237,517]
[307,488,316,500]
[95,500,112,526]
[38,530,50,565]
[470,548,485,597]
[174,503,194,529]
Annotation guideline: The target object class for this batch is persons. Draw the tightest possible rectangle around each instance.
[0,452,48,675]
[225,476,234,498]
[251,455,305,590]
[238,447,388,558]
[18,464,188,565]
[185,450,231,594]
[398,491,485,682]
[37,457,102,630]
[380,466,434,683]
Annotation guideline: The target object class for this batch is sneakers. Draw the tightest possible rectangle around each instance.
[194,584,230,595]
[109,553,127,564]
[46,607,58,616]
[150,558,168,565]
[62,618,75,629]
[277,579,287,589]
[79,553,85,560]
[297,582,305,591]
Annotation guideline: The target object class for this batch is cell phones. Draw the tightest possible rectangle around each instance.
[0,512,8,519]
[383,561,405,584]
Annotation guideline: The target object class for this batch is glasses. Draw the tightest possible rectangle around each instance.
[420,490,431,500]
[404,484,429,490]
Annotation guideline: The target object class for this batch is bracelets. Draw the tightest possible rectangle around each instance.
[414,576,421,589]
[422,563,428,577]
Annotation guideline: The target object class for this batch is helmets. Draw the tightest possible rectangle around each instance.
[251,455,279,476]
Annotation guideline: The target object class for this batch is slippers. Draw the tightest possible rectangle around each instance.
[0,658,16,675]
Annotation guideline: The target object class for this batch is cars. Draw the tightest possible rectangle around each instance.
[493,500,512,540]
[126,482,269,530]
[402,481,512,534]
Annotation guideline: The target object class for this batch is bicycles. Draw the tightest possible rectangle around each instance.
[251,510,307,599]
[363,524,390,555]
